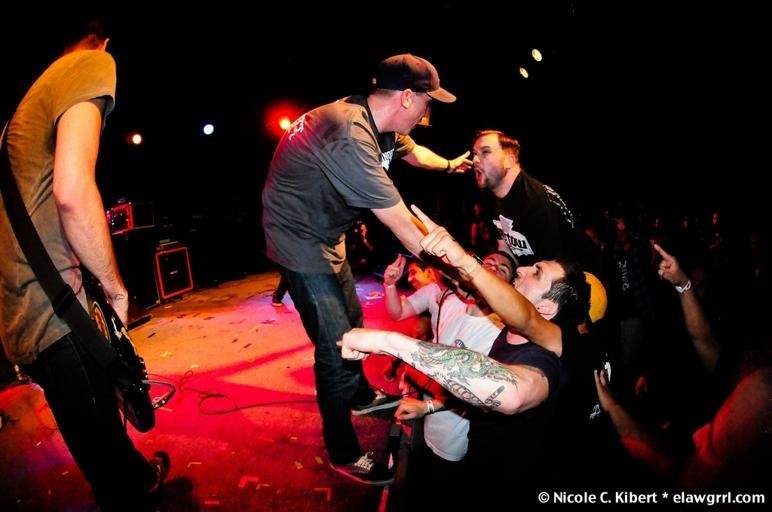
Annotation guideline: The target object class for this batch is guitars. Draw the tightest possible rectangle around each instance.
[81,264,155,433]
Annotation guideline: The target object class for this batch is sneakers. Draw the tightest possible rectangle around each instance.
[329,450,395,485]
[151,451,170,493]
[351,389,403,416]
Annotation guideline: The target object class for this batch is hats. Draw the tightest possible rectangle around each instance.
[371,53,456,104]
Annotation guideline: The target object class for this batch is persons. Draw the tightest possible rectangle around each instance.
[336,260,591,512]
[0,13,194,511]
[408,255,446,294]
[473,129,575,267]
[648,245,772,403]
[593,363,771,503]
[629,207,699,260]
[606,215,642,327]
[384,251,517,356]
[584,228,616,298]
[262,53,474,484]
[699,211,721,281]
[410,203,613,456]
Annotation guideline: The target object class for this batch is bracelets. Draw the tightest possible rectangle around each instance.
[444,160,452,173]
[673,279,692,293]
